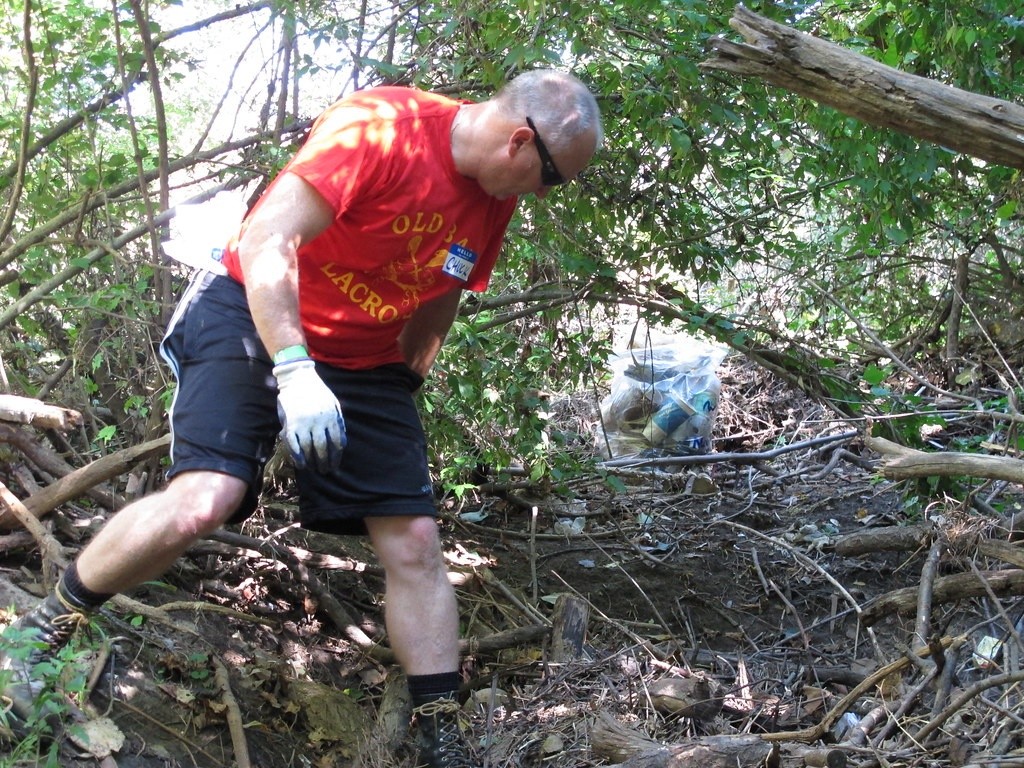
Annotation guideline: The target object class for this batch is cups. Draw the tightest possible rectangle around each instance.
[607,372,720,454]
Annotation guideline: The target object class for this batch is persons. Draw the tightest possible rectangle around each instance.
[0,70,605,768]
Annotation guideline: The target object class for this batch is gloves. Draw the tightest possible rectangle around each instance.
[271,360,348,471]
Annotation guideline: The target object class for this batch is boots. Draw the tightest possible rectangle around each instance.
[0,579,104,684]
[414,689,481,768]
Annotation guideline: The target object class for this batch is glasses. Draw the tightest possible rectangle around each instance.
[525,116,566,187]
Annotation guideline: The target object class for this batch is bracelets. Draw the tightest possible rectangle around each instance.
[273,345,309,366]
